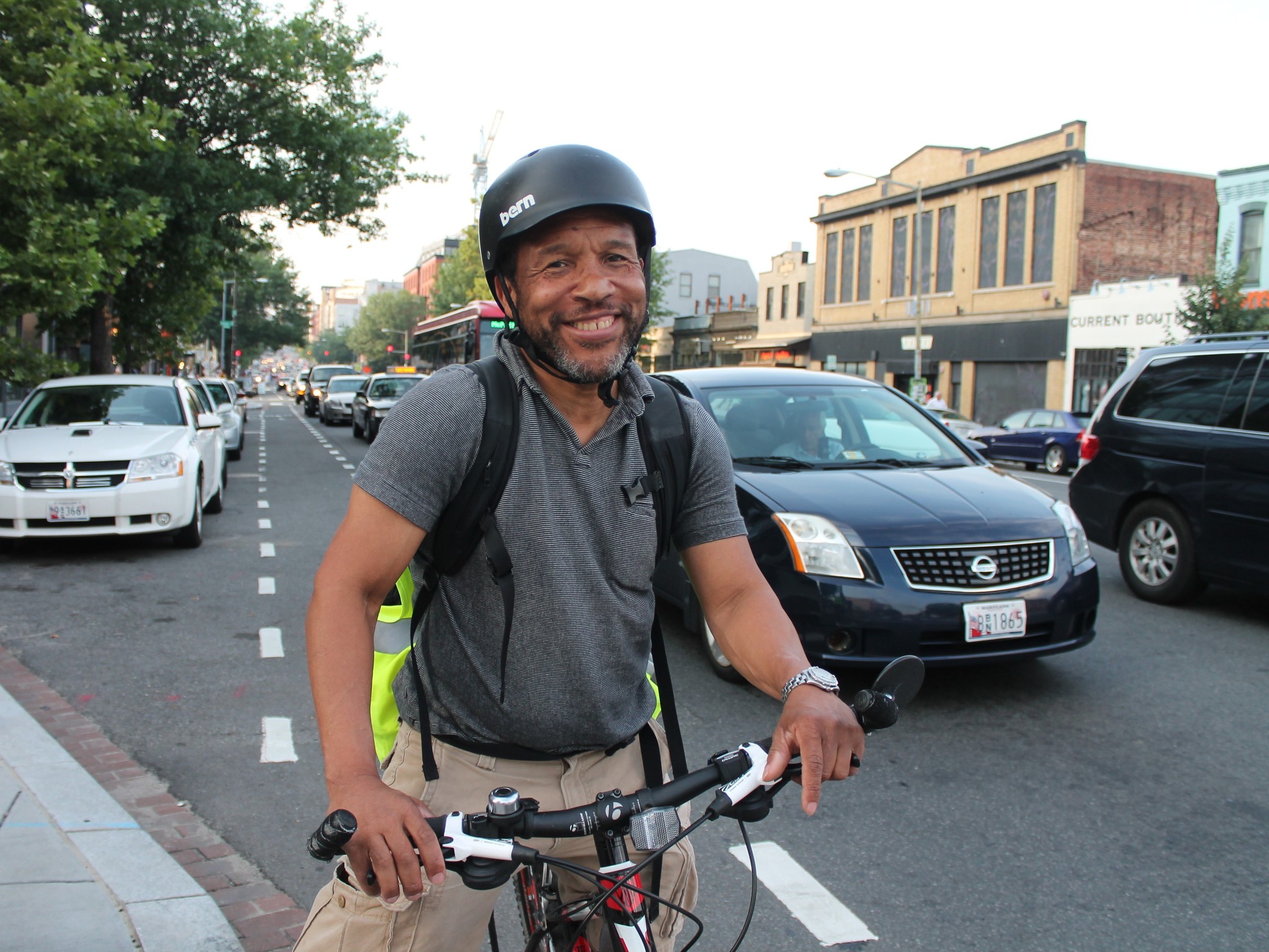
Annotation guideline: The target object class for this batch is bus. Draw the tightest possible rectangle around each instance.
[412,300,516,374]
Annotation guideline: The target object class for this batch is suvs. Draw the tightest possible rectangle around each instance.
[1067,330,1269,614]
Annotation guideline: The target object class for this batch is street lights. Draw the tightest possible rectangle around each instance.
[221,277,270,371]
[381,328,409,367]
[824,168,924,384]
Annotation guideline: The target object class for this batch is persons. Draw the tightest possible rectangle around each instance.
[771,407,845,463]
[292,145,865,952]
[920,390,949,410]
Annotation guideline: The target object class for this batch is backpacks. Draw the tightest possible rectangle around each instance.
[368,353,690,768]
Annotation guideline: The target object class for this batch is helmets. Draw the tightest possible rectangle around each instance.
[478,144,658,276]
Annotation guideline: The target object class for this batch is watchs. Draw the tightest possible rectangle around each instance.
[783,667,840,704]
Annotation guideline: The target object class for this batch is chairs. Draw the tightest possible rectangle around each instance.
[724,403,789,457]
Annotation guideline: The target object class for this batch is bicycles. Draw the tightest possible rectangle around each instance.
[303,655,927,952]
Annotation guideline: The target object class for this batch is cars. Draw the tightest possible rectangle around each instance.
[0,370,250,548]
[629,365,1103,687]
[274,363,429,444]
[920,403,1086,475]
